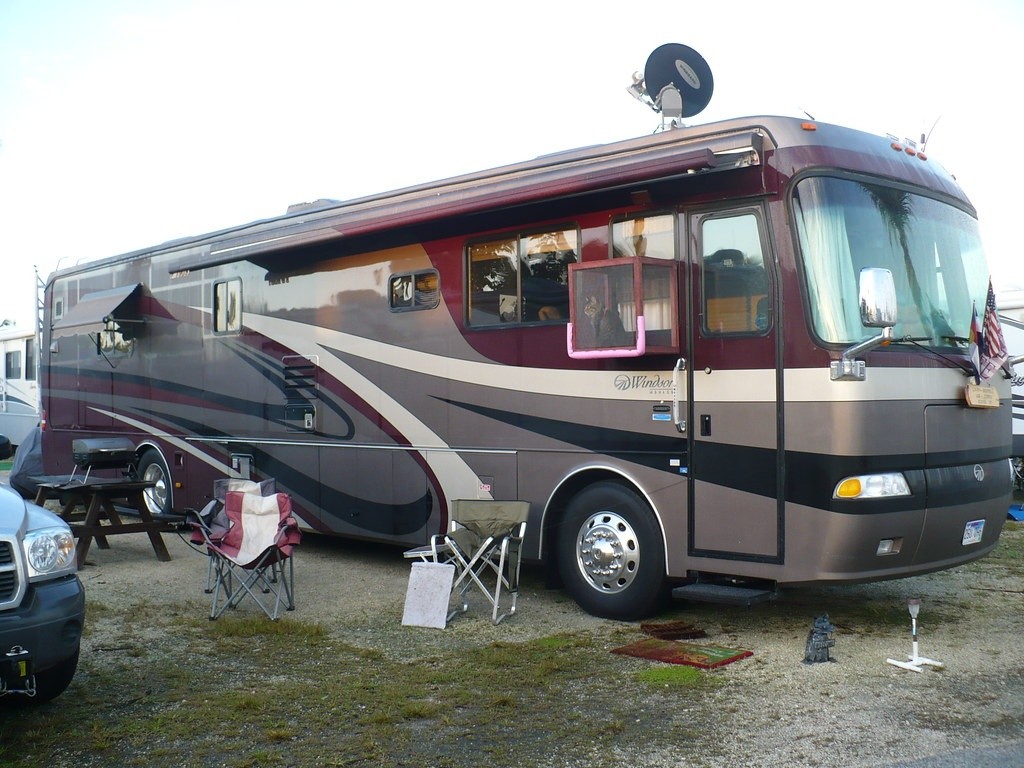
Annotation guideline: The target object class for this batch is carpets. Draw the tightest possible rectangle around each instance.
[610,638,753,670]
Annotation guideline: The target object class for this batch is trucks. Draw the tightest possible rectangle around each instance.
[1,325,42,449]
[39,115,1024,621]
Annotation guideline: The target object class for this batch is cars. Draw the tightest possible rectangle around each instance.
[0,483,85,718]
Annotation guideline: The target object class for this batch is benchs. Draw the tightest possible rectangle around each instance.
[113,505,186,522]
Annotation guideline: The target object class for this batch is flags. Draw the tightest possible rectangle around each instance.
[979,282,1008,381]
[969,303,983,385]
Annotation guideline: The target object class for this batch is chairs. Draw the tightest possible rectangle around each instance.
[185,477,302,622]
[430,497,530,627]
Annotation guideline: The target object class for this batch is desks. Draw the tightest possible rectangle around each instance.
[28,475,171,571]
[405,545,451,564]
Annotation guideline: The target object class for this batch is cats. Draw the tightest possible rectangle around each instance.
[584,295,626,347]
